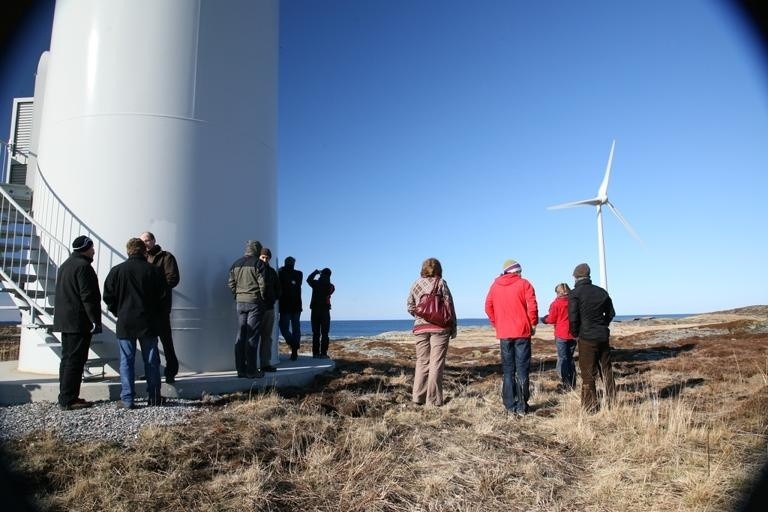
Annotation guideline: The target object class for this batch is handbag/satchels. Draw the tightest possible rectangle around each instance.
[414,276,456,329]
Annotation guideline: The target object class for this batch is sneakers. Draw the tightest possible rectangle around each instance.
[57,397,93,410]
[115,399,133,409]
[136,373,176,384]
[320,354,330,359]
[237,370,264,379]
[290,353,298,361]
[261,364,277,372]
[147,396,166,406]
[313,353,320,358]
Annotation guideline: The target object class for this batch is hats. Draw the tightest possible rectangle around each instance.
[260,247,271,258]
[502,259,521,273]
[572,263,591,278]
[72,235,93,252]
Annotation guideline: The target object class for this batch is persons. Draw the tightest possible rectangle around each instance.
[259,248,282,373]
[137,230,181,383]
[277,256,303,361]
[540,282,577,392]
[306,267,336,358]
[405,256,458,407]
[568,262,616,415]
[228,239,270,377]
[485,259,539,414]
[103,238,168,408]
[53,236,101,410]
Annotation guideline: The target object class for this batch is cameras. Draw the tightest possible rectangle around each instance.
[318,269,322,274]
